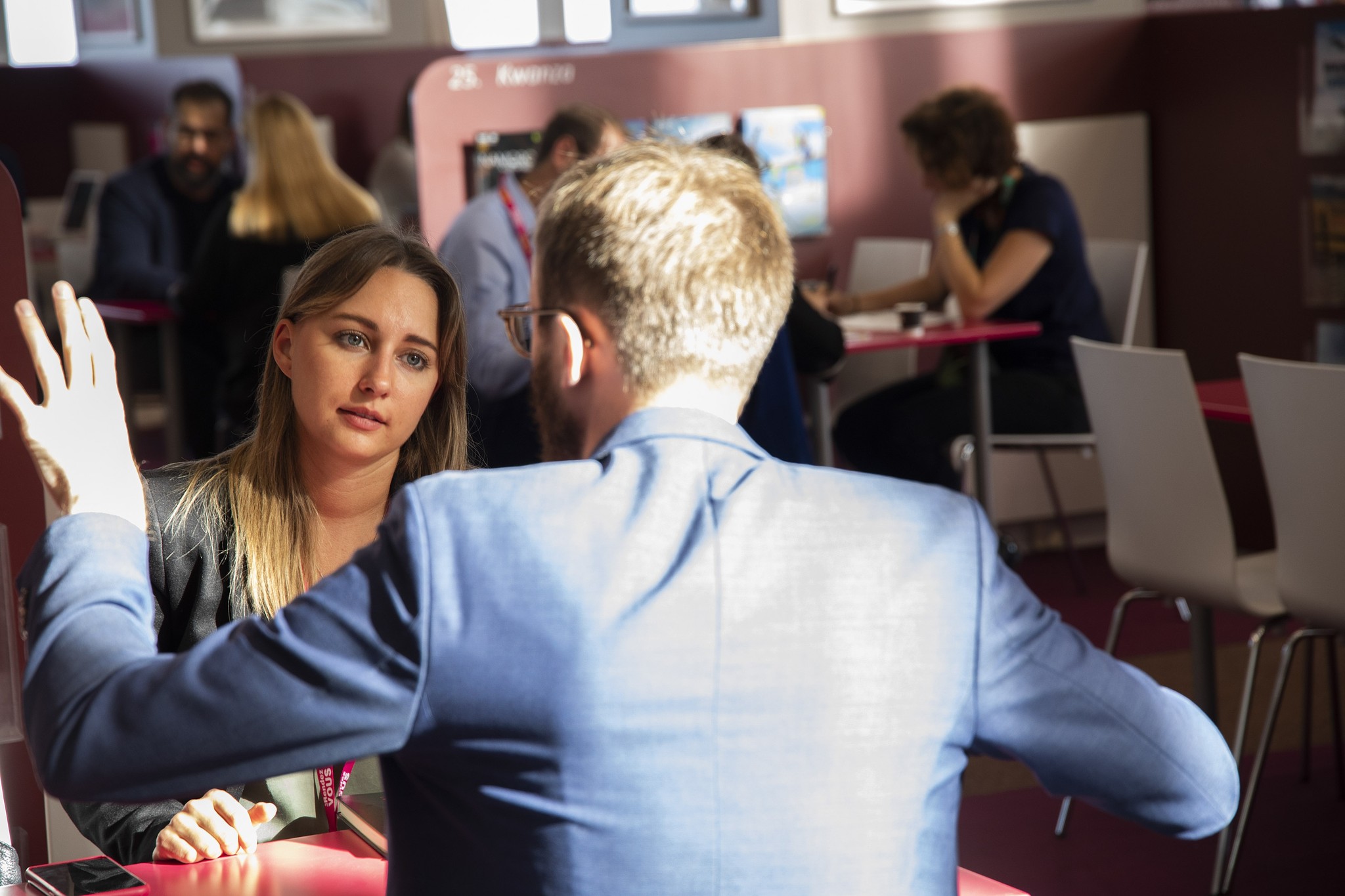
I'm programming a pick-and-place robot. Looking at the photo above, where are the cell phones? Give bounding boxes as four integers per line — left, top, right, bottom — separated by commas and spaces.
24, 855, 151, 896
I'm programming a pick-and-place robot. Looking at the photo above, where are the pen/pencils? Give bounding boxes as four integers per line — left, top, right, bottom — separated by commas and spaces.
821, 263, 835, 301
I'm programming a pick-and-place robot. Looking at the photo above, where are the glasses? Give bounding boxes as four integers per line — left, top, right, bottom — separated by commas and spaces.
496, 301, 594, 362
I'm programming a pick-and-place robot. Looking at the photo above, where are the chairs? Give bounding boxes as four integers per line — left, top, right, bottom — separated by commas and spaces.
802, 237, 935, 468
1052, 335, 1345, 896
945, 233, 1147, 592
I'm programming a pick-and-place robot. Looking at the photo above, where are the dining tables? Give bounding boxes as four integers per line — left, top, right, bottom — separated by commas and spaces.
0, 827, 1040, 896
812, 307, 1043, 536
91, 297, 192, 466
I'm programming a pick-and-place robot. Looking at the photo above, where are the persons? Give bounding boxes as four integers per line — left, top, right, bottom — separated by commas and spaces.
12, 221, 467, 863
177, 89, 386, 464
433, 103, 627, 467
688, 132, 848, 469
818, 82, 1128, 496
0, 137, 1242, 896
89, 78, 246, 460
